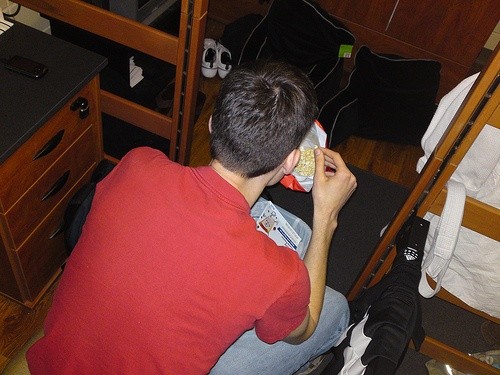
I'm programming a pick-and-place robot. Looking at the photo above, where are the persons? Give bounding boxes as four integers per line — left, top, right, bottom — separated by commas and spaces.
25, 60, 357, 375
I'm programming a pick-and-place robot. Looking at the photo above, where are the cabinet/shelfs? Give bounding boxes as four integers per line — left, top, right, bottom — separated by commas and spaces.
0, 15, 109, 310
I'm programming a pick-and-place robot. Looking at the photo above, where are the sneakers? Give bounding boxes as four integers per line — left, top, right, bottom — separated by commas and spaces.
200, 38, 217, 78
216, 42, 232, 79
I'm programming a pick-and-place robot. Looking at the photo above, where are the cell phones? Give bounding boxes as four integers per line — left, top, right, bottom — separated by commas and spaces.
5, 55, 47, 78
